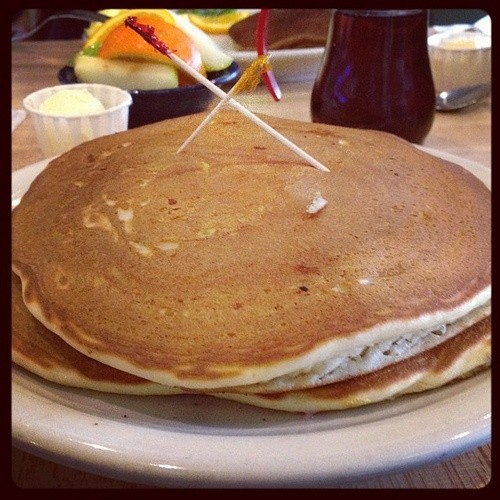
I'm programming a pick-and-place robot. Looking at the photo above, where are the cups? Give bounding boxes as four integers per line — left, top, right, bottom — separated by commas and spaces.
23, 83, 133, 158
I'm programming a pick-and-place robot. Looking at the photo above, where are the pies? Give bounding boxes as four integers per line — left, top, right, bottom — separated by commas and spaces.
11, 109, 491, 410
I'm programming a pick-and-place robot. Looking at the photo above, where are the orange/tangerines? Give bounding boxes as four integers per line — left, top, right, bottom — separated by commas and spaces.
83, 9, 207, 87
188, 11, 250, 34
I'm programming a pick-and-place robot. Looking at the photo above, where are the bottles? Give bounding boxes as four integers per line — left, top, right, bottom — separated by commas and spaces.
310, 8, 436, 144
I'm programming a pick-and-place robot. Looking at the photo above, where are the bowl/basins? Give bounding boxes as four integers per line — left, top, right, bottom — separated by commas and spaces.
423, 31, 491, 110
55, 61, 239, 126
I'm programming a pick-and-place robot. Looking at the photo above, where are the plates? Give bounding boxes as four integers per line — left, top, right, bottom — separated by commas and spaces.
223, 50, 324, 82
12, 151, 492, 486
